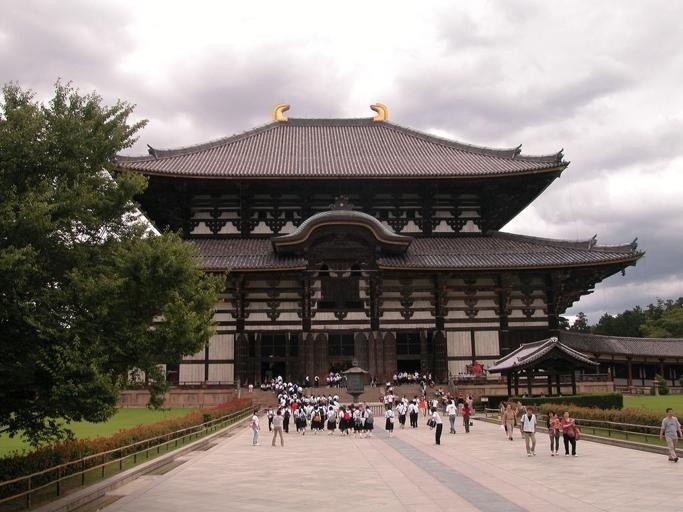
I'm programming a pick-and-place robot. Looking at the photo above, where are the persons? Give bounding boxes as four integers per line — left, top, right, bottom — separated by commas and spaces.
549, 412, 561, 434
557, 411, 579, 457
245, 368, 486, 449
515, 400, 528, 439
520, 406, 538, 457
659, 407, 683, 463
501, 403, 520, 443
545, 410, 561, 457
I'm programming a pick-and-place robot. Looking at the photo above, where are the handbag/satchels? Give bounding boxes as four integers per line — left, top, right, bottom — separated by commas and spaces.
344, 412, 349, 420
427, 419, 436, 428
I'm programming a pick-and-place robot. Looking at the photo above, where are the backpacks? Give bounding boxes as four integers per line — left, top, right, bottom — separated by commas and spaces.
355, 412, 361, 424
312, 410, 321, 422
366, 411, 374, 424
